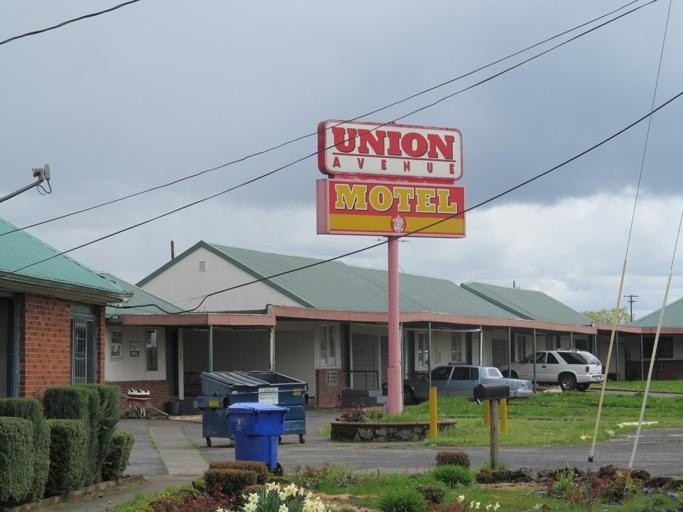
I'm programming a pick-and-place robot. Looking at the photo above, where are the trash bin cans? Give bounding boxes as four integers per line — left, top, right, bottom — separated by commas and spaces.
196, 368, 309, 447
224, 402, 290, 477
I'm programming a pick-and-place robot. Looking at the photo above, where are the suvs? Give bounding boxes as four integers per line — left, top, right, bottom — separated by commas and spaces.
497, 348, 607, 392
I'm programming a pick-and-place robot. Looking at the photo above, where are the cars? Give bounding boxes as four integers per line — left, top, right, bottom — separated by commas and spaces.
381, 361, 535, 407
